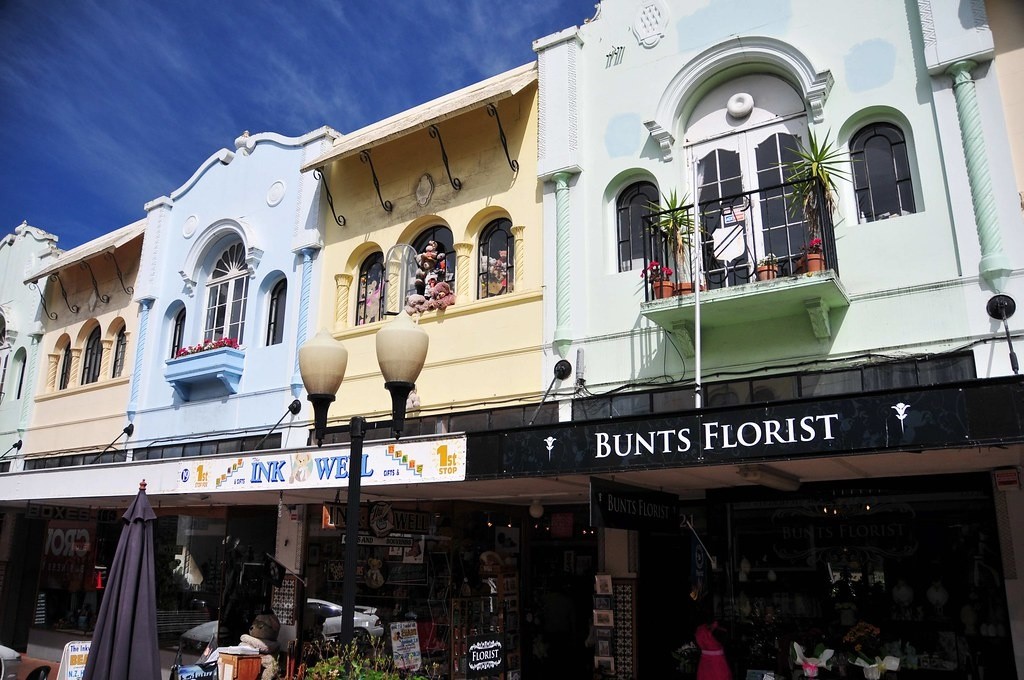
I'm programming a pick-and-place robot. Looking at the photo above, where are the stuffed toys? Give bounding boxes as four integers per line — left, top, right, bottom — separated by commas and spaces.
238, 610, 281, 680
404, 240, 456, 316
365, 275, 384, 324
481, 250, 508, 286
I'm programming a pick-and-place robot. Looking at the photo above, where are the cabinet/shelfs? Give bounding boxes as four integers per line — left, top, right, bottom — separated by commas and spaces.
451, 571, 521, 680
218, 653, 263, 680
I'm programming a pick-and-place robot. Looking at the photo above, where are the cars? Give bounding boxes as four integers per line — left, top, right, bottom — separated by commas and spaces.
180, 597, 384, 662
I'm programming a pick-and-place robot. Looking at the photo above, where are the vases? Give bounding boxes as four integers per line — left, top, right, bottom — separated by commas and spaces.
806, 254, 827, 272
757, 265, 778, 281
653, 281, 675, 299
164, 347, 245, 385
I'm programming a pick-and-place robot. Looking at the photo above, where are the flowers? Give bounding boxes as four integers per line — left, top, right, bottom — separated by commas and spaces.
640, 261, 673, 284
294, 635, 443, 680
757, 252, 778, 267
802, 238, 823, 272
175, 336, 240, 359
843, 622, 880, 665
671, 642, 700, 673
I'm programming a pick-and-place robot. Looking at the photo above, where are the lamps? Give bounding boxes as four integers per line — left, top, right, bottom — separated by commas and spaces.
529, 499, 544, 518
736, 464, 800, 491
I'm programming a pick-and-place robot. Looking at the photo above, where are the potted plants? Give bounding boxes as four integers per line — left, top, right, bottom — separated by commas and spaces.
640, 187, 713, 295
770, 124, 864, 273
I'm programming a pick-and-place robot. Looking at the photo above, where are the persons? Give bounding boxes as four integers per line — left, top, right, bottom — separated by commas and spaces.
695, 616, 733, 680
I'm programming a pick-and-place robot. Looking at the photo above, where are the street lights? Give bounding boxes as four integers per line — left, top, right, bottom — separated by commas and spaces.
297, 311, 429, 680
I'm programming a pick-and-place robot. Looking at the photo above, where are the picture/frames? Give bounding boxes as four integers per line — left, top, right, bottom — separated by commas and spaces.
595, 656, 614, 672
595, 575, 613, 594
593, 609, 614, 626
